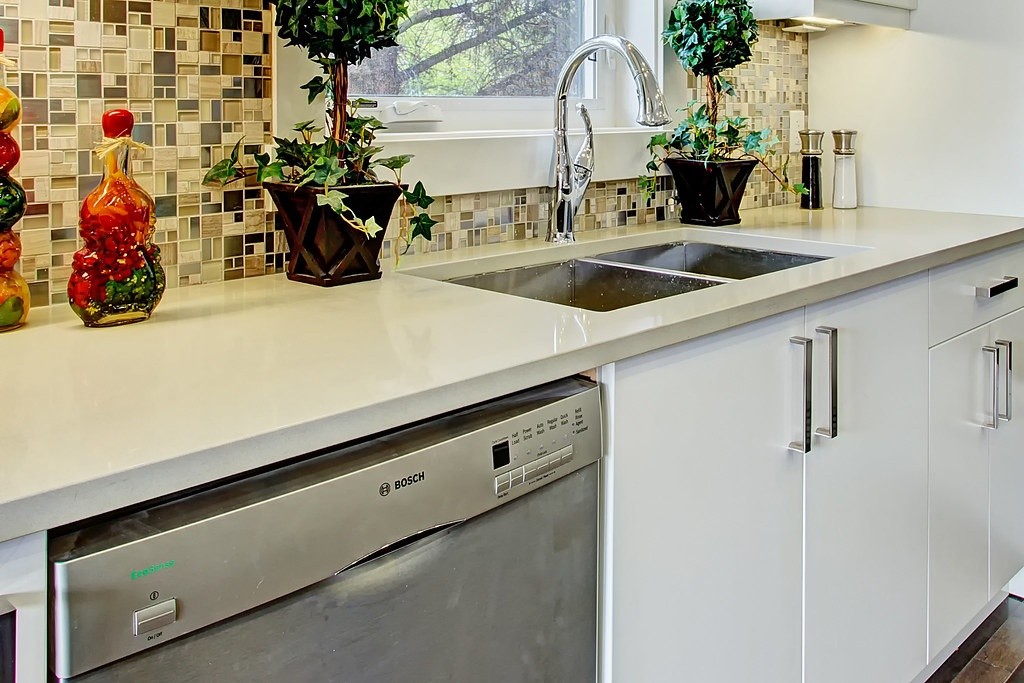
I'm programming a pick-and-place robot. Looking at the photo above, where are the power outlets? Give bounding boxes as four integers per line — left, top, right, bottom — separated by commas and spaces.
789, 110, 805, 153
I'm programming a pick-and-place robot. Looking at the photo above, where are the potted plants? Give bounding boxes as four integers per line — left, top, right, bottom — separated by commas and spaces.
637, 0, 810, 226
202, 1, 438, 289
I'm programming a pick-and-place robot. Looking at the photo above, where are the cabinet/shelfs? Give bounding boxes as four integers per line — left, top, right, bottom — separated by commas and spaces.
929, 241, 1024, 683
599, 271, 928, 682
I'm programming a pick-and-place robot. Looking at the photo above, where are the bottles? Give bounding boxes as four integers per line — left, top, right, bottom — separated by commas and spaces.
798, 128, 824, 209
66, 110, 168, 327
830, 129, 860, 210
0, 28, 31, 333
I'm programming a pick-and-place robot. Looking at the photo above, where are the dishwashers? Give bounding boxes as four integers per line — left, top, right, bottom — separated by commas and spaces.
48, 378, 602, 683
